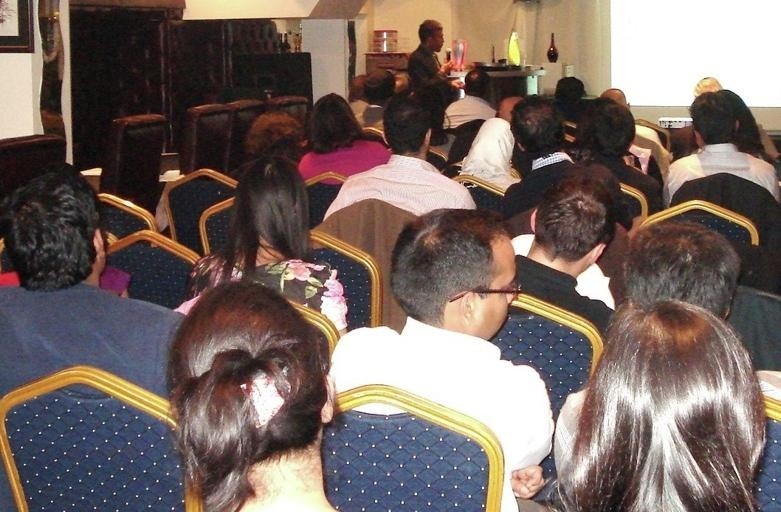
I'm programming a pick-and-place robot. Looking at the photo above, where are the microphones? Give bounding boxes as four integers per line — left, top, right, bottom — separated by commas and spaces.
445, 48, 452, 76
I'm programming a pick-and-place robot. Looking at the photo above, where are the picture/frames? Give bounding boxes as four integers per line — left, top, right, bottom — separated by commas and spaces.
0, 0, 34, 53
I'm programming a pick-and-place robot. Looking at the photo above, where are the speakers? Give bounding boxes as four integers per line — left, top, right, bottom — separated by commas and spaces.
232, 52, 313, 110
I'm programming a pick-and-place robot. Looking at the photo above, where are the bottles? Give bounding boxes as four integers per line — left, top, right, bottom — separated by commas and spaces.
233, 28, 301, 54
547, 34, 558, 64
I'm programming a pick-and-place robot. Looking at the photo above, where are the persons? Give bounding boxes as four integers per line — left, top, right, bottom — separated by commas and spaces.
0, 184, 133, 299
170, 279, 346, 510
227, 70, 781, 343
406, 19, 468, 94
549, 219, 781, 490
0, 158, 193, 511
562, 297, 768, 509
322, 205, 561, 512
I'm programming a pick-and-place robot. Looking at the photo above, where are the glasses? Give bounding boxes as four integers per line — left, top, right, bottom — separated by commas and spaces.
449, 271, 520, 306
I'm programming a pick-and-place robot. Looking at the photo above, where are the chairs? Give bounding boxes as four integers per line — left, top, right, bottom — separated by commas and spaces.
1, 95, 781, 512
